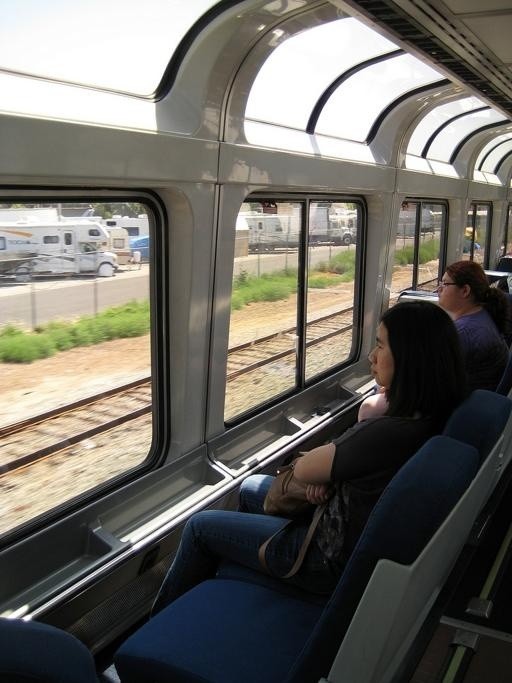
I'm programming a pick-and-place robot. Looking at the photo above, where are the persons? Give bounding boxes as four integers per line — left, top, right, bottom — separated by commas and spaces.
150, 300, 472, 619
357, 260, 511, 423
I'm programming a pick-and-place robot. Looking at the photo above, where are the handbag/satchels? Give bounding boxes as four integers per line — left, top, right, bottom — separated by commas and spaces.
262, 456, 331, 515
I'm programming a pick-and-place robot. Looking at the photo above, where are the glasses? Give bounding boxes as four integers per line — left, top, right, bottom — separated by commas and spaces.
438, 281, 458, 288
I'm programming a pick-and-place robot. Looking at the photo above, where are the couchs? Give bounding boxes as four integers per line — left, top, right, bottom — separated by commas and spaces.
212, 250, 511, 614
110, 435, 503, 680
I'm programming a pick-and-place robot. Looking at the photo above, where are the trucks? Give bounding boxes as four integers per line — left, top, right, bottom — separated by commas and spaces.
0, 206, 149, 281
246, 204, 437, 252
